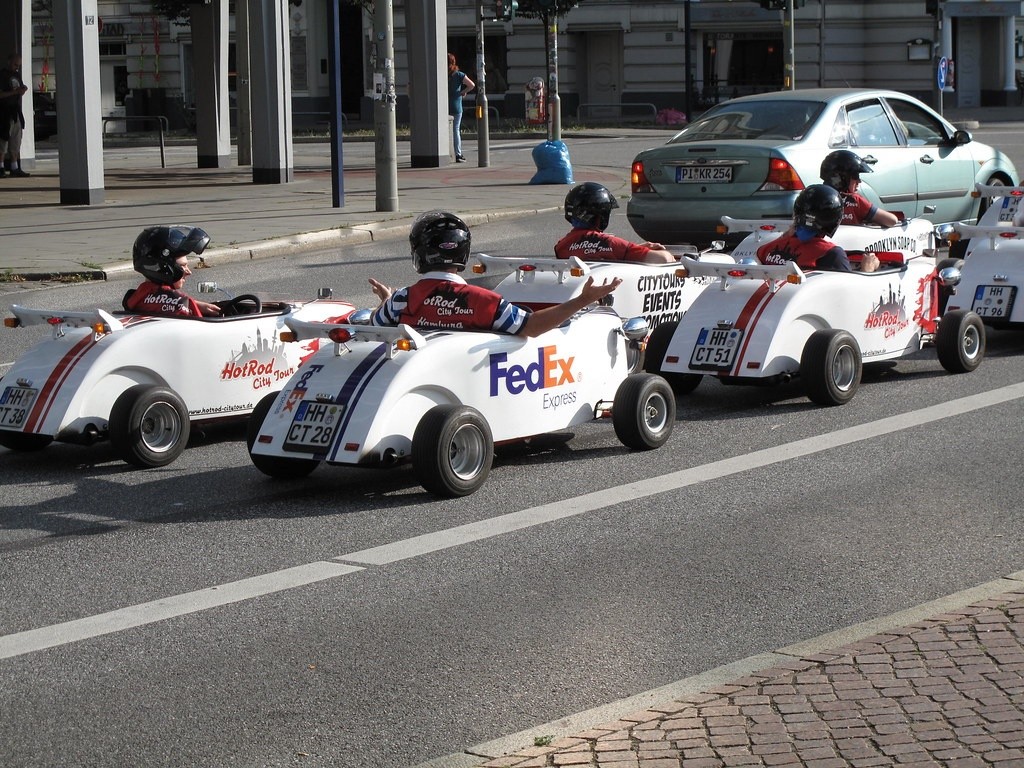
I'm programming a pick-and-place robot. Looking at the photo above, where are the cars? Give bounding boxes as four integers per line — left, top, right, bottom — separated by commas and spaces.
627, 88, 1019, 241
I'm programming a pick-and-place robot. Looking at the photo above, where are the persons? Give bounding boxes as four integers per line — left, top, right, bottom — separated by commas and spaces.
448, 53, 475, 163
369, 209, 623, 338
122, 226, 224, 319
753, 184, 880, 273
554, 182, 666, 262
820, 150, 898, 228
0, 54, 30, 177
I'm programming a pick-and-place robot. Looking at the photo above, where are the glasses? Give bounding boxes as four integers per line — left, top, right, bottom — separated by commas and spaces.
850, 172, 859, 181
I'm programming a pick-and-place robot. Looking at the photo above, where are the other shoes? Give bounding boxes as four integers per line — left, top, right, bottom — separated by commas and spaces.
456, 154, 467, 163
0, 167, 7, 178
9, 168, 31, 177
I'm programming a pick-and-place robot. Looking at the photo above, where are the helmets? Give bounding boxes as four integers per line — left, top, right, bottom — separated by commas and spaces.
821, 150, 875, 192
133, 227, 186, 285
794, 185, 844, 239
565, 182, 619, 228
410, 213, 472, 273
